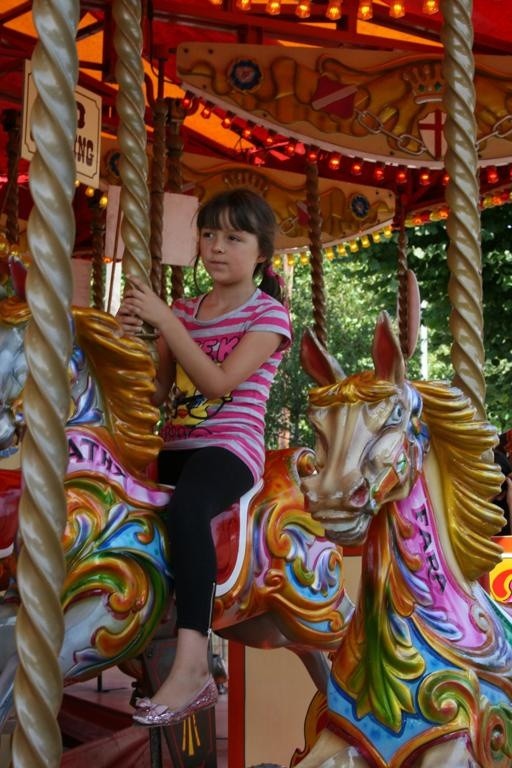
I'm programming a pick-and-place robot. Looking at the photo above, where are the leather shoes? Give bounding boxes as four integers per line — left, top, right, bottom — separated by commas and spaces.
132, 673, 221, 728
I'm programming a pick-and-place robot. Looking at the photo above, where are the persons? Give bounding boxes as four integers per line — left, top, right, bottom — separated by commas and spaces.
111, 188, 297, 727
489, 432, 512, 536
